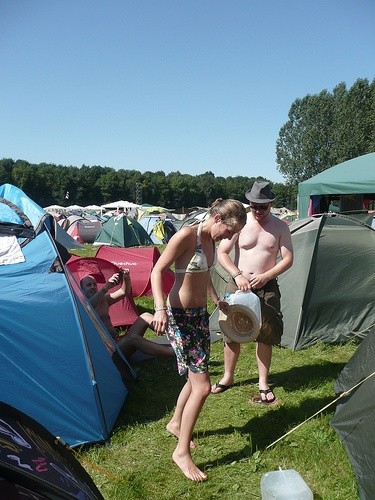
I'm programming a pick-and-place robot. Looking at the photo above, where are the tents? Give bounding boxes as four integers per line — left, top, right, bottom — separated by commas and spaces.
0, 151, 375, 500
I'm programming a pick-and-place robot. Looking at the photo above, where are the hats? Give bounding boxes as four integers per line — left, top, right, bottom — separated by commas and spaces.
218, 303, 260, 344
245, 180, 277, 204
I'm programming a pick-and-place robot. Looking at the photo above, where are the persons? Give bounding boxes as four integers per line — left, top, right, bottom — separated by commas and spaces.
80, 269, 176, 362
149, 198, 247, 481
211, 180, 294, 403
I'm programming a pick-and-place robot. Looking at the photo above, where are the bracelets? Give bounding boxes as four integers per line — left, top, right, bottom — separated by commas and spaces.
233, 271, 242, 279
154, 306, 167, 311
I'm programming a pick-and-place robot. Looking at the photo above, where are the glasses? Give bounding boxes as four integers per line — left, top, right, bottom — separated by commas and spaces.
250, 204, 269, 210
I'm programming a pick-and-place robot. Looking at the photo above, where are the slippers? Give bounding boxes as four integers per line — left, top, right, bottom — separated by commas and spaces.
258, 388, 275, 403
210, 378, 231, 394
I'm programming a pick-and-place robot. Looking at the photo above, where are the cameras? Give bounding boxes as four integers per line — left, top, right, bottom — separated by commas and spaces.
115, 271, 123, 285
